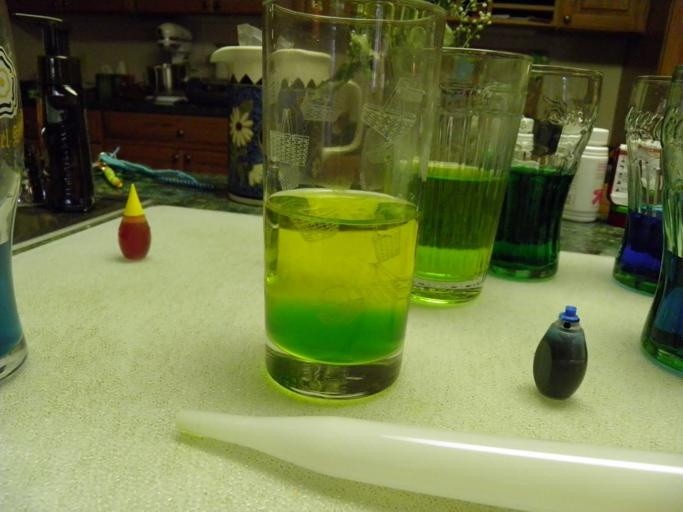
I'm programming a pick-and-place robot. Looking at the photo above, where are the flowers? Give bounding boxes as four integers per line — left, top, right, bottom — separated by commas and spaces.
311, 0, 492, 90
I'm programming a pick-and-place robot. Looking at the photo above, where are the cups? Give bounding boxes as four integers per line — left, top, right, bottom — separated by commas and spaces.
211, 37, 362, 207
260, 0, 445, 402
387, 46, 534, 308
483, 53, 604, 283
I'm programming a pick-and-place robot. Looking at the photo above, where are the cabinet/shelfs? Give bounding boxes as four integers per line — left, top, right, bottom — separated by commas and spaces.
431, 0, 673, 37
18, 108, 229, 183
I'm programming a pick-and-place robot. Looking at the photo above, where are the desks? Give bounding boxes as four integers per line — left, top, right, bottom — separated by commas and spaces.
0, 190, 683, 512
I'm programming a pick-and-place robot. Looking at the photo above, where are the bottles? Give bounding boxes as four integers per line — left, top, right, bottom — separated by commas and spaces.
612, 72, 671, 294
531, 303, 589, 399
116, 185, 152, 262
12, 24, 99, 212
566, 122, 608, 222
0, 46, 30, 386
643, 68, 683, 378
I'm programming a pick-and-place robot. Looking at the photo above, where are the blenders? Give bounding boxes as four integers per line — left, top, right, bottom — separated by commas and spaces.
146, 23, 192, 108
